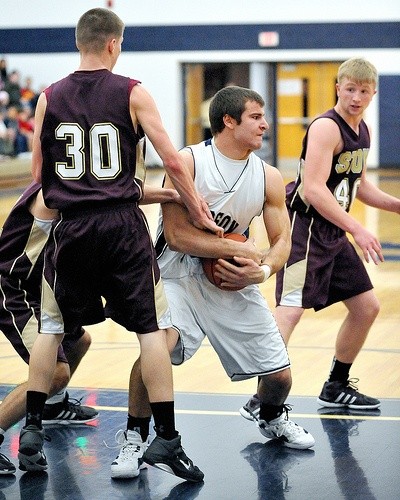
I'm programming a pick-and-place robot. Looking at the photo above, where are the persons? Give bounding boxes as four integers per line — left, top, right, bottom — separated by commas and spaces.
238, 56, 400, 426
107, 91, 315, 475
20, 10, 224, 481
0, 60, 205, 474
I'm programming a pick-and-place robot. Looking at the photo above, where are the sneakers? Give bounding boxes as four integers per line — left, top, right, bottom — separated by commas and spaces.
39, 393, 99, 422
143, 432, 204, 481
0, 434, 15, 475
42, 424, 97, 448
161, 482, 203, 500
317, 377, 380, 410
241, 440, 315, 480
110, 430, 148, 478
241, 395, 262, 421
256, 411, 315, 449
317, 408, 381, 436
18, 425, 49, 470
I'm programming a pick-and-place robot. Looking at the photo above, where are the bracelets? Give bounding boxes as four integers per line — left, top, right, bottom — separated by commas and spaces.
260, 265, 271, 283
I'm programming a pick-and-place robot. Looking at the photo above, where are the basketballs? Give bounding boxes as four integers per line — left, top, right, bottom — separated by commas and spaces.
202, 233, 256, 291
